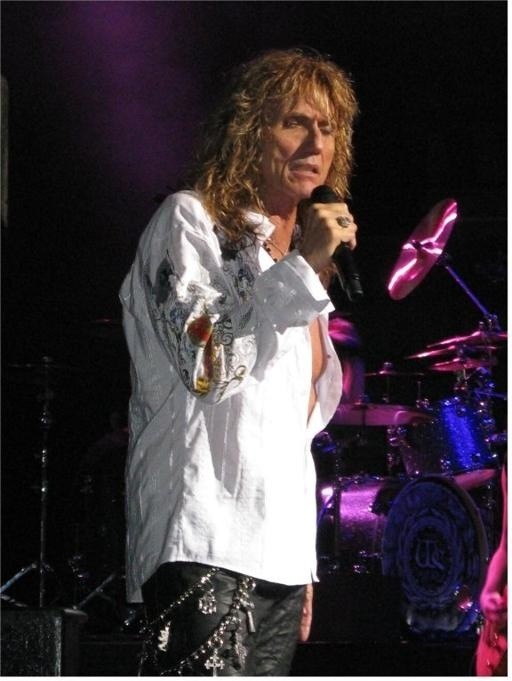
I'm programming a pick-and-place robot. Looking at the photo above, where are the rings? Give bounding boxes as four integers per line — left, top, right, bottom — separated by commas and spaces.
336, 215, 351, 228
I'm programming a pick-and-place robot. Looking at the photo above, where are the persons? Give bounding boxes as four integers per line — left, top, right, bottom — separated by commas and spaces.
327, 319, 363, 405
116, 46, 358, 676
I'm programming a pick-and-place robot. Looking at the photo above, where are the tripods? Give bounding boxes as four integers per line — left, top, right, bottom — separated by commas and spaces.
78, 524, 125, 607
0, 402, 64, 610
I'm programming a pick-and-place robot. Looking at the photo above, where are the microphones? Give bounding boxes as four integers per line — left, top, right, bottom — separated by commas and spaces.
311, 184, 366, 304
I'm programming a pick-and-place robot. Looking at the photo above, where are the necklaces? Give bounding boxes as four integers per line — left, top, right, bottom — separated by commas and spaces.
266, 238, 289, 257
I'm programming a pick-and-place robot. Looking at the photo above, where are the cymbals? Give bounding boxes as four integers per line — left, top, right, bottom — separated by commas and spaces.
408, 330, 507, 372
366, 369, 403, 377
337, 401, 410, 427
384, 199, 458, 299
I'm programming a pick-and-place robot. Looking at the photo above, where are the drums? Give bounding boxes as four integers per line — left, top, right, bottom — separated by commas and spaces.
434, 393, 498, 471
381, 475, 503, 645
315, 476, 390, 559
